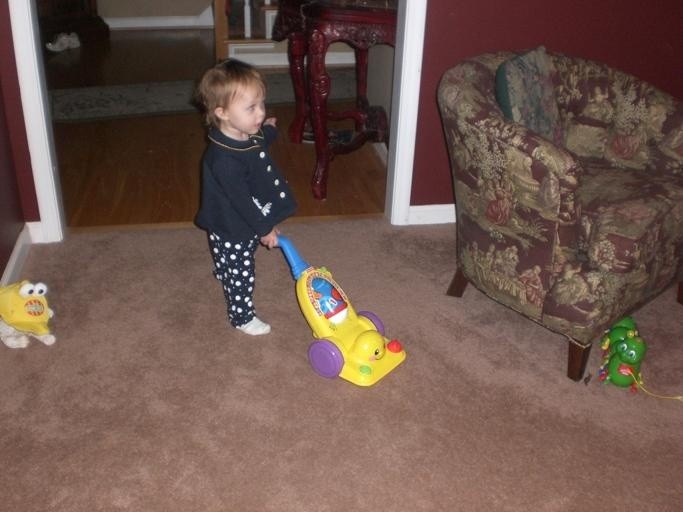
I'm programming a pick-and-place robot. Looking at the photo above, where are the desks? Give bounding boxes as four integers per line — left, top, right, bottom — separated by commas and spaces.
286, 6, 397, 202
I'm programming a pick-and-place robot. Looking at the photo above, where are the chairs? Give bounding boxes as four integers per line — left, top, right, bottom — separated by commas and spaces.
434, 51, 683, 383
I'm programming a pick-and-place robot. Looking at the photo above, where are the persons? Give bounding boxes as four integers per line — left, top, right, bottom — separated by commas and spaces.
194, 58, 296, 336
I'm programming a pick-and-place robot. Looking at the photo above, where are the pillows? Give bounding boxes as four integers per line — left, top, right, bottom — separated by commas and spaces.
494, 43, 563, 149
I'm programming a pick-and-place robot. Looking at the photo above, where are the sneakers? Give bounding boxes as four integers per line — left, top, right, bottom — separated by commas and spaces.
235, 315, 271, 336
